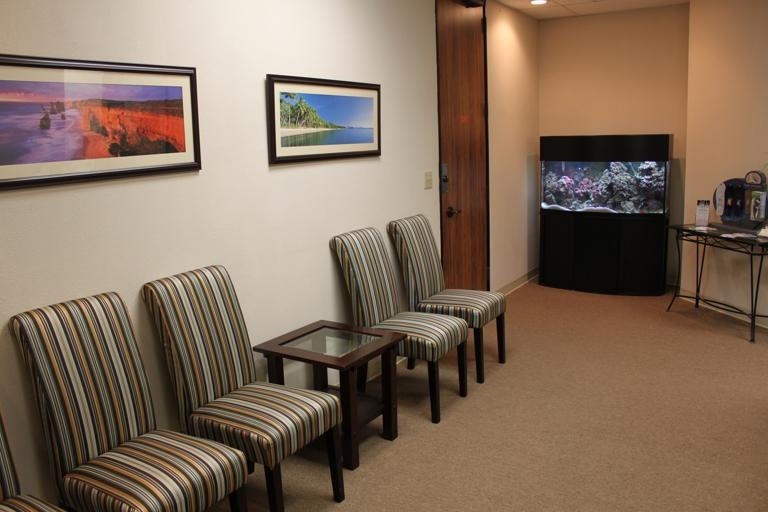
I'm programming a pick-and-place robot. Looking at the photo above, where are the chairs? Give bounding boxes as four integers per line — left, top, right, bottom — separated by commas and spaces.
142, 264, 346, 510
9, 291, 249, 512
330, 212, 509, 424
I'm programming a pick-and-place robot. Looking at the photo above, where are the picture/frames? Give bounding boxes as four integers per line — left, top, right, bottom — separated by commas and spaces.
264, 71, 383, 165
0, 50, 202, 188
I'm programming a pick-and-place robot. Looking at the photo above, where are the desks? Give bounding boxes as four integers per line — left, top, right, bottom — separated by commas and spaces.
666, 223, 768, 344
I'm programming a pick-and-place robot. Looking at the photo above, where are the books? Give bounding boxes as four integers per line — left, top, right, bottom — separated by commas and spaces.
749, 190, 766, 222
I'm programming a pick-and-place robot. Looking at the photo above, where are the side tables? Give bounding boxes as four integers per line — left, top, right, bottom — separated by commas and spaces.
251, 318, 408, 470
